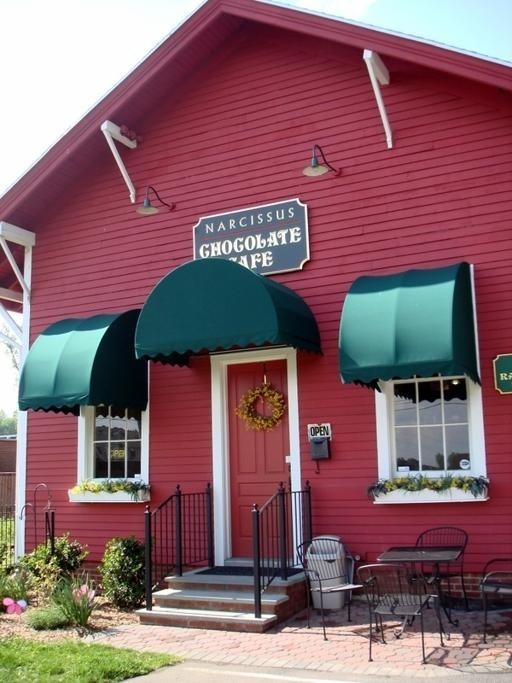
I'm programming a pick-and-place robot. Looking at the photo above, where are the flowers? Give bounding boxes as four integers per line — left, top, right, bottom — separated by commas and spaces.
367, 473, 491, 500
235, 382, 287, 430
71, 478, 151, 500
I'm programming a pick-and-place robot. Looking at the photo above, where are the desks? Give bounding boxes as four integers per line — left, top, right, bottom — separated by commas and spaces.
375, 544, 464, 637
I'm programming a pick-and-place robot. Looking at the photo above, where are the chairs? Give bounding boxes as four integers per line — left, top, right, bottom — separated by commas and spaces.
354, 563, 450, 666
478, 557, 512, 644
296, 537, 383, 640
407, 526, 468, 628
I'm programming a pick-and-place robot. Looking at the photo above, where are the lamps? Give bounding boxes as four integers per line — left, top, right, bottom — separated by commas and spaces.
301, 144, 342, 178
135, 185, 176, 215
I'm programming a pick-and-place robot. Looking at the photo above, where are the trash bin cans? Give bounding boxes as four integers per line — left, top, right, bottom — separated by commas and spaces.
305, 535, 345, 611
